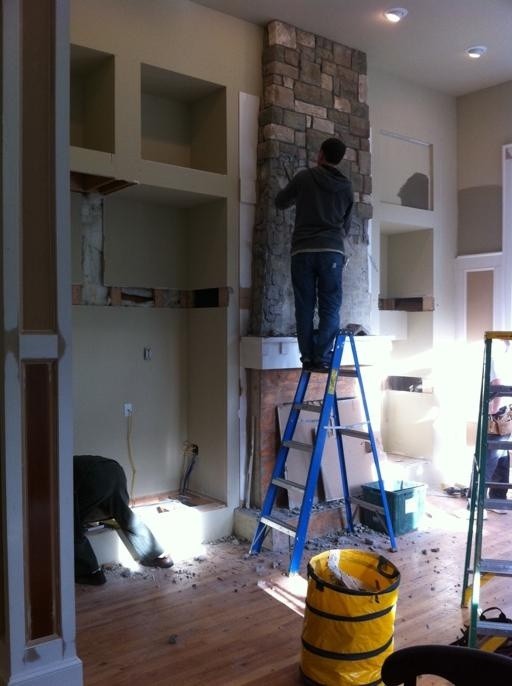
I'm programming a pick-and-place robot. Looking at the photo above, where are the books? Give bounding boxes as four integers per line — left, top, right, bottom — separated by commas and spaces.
348, 323, 369, 335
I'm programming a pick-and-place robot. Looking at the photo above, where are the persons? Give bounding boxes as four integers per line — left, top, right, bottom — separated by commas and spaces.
273, 137, 356, 371
467, 336, 512, 521
74, 453, 173, 587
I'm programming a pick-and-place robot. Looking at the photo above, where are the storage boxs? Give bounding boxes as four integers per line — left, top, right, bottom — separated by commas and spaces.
359, 479, 428, 536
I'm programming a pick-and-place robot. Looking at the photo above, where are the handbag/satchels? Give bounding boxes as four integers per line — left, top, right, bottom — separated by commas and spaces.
486, 406, 511, 438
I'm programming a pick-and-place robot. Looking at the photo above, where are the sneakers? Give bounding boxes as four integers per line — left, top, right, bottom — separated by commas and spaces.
139, 556, 173, 569
302, 359, 331, 372
78, 571, 106, 585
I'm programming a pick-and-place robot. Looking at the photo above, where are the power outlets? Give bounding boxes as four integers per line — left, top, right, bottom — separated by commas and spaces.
121, 402, 135, 418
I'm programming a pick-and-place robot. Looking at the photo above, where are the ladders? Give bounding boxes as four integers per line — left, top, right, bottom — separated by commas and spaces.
248, 329, 397, 578
460, 330, 512, 650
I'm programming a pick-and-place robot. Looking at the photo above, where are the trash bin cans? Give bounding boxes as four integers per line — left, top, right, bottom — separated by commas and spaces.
298, 549, 400, 686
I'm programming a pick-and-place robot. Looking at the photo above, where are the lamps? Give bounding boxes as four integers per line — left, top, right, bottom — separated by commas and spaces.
465, 45, 487, 60
384, 6, 409, 23
